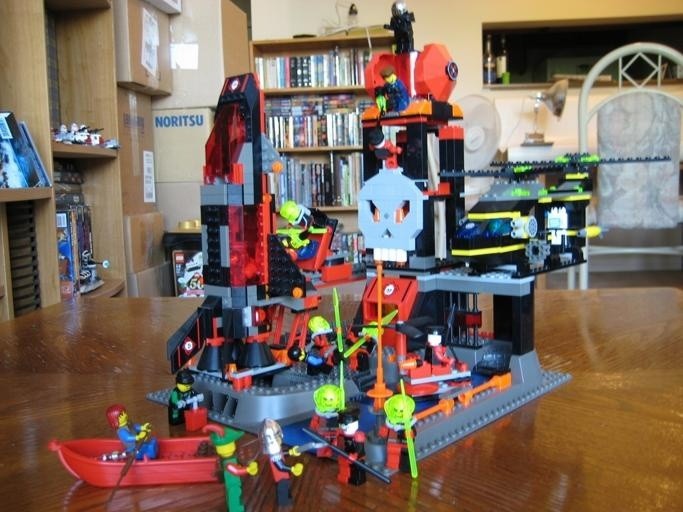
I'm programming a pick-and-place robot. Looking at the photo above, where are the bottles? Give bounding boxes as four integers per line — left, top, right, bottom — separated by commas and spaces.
496, 36, 508, 83
483, 34, 497, 85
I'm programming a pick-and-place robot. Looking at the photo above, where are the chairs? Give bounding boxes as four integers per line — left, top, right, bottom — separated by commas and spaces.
562, 39, 683, 292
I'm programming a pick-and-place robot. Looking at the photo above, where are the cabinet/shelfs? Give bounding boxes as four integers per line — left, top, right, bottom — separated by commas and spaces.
0, 2, 130, 324
249, 32, 402, 267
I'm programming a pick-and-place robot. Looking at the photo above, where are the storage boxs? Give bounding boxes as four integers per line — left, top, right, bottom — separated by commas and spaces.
114, 1, 250, 110
113, 85, 216, 296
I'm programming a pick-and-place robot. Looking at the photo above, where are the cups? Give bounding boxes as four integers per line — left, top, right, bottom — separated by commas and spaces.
177, 219, 202, 231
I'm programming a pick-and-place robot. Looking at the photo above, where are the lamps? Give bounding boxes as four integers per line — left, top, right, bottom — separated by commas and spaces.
518, 77, 571, 149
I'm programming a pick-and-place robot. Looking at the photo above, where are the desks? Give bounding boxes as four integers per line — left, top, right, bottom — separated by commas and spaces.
0, 291, 681, 511
504, 145, 566, 165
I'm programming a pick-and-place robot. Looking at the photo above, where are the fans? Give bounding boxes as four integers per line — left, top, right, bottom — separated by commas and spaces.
428, 92, 503, 179
